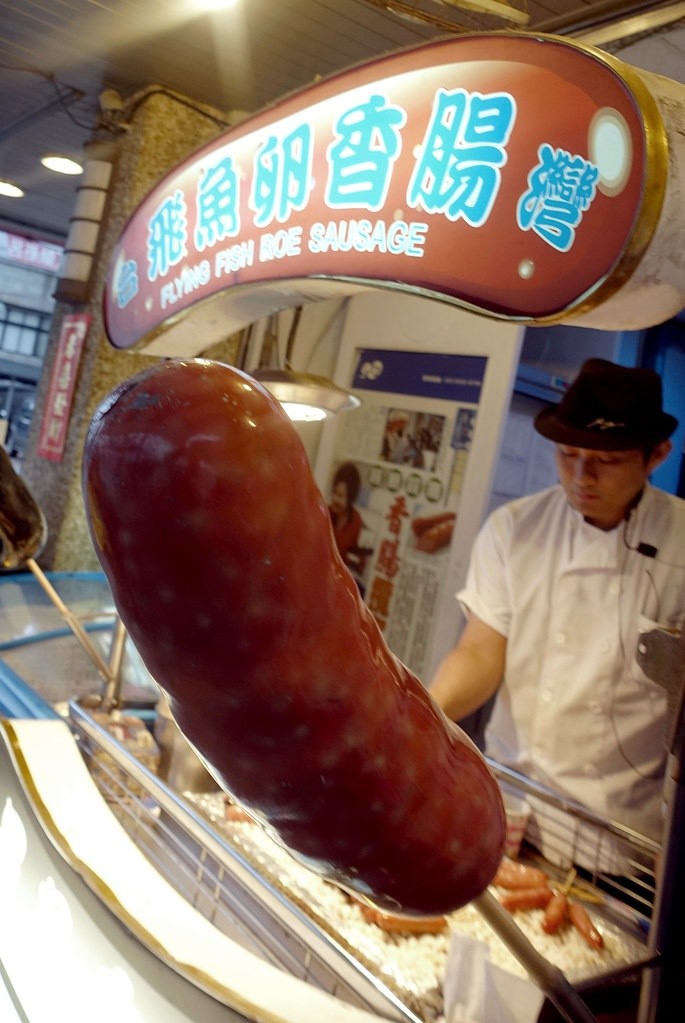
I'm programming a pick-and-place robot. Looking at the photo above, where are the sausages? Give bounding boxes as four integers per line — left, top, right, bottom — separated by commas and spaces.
487, 840, 616, 967
74, 357, 512, 921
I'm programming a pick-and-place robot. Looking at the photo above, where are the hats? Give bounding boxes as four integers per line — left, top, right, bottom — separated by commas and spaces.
534, 358, 678, 451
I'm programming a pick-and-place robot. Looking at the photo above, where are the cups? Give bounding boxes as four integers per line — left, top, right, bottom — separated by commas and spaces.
501, 792, 531, 862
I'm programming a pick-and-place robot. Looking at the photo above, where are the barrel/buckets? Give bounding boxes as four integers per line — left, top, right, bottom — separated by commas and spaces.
152, 702, 220, 797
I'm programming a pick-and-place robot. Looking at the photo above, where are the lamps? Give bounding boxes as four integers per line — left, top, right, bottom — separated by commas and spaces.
241, 304, 366, 428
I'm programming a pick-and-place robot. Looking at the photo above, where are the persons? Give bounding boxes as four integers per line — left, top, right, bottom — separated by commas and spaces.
325, 462, 360, 565
415, 360, 685, 915
